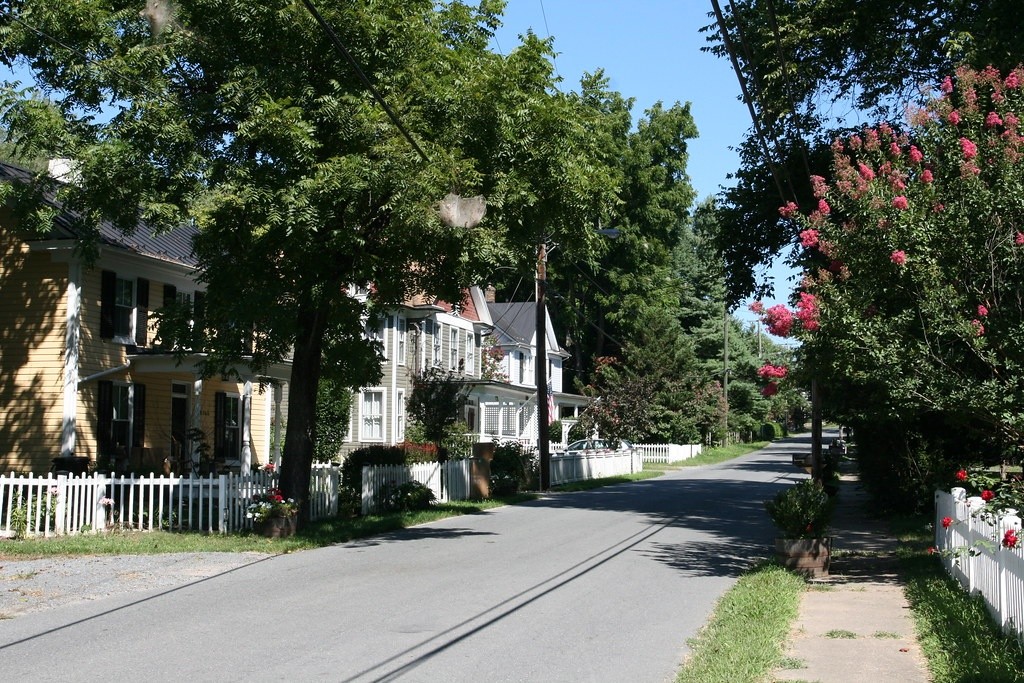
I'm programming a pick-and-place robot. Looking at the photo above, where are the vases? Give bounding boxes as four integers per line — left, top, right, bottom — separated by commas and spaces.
263, 518, 297, 537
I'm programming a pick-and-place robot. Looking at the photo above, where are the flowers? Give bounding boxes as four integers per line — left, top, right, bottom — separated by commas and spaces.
957, 470, 967, 481
1003, 530, 1017, 547
981, 489, 996, 502
942, 516, 952, 529
245, 489, 297, 522
928, 546, 933, 552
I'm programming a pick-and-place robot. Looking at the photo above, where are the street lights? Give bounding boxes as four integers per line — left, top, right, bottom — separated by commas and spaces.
535, 227, 620, 491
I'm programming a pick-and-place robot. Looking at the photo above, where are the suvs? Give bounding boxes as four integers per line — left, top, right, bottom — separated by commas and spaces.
555, 437, 633, 454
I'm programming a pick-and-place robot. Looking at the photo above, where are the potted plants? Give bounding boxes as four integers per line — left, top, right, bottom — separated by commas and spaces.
188, 428, 218, 478
388, 479, 435, 512
489, 439, 527, 497
763, 479, 836, 579
